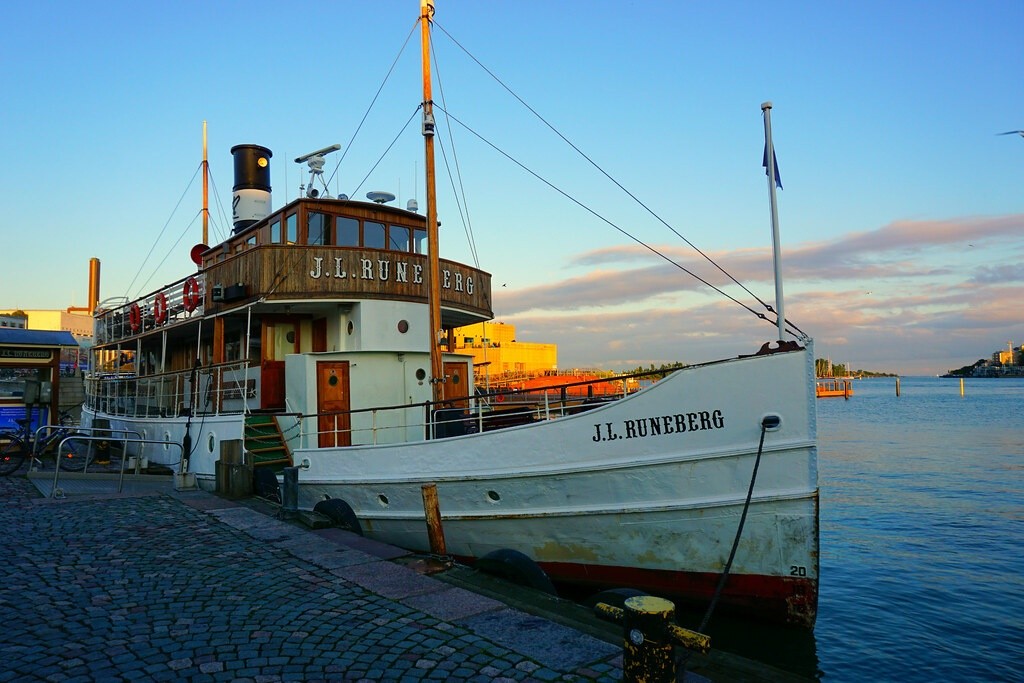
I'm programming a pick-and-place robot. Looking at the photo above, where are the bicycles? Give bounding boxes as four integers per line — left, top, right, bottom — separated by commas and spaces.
0, 400, 96, 476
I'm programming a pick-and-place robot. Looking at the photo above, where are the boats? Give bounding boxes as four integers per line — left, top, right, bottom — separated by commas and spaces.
78, 0, 821, 636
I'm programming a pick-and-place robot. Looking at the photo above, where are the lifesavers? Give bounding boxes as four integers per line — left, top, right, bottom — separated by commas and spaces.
154, 292, 166, 323
130, 305, 141, 331
182, 278, 199, 312
497, 395, 504, 402
